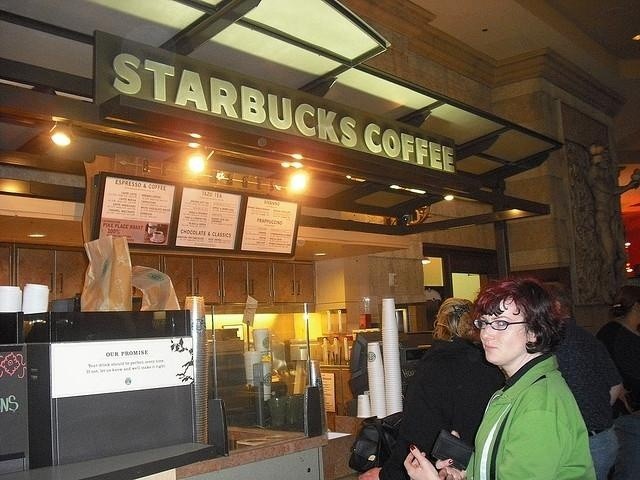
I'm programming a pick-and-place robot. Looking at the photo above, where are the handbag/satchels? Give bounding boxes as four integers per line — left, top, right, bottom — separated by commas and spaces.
348, 413, 402, 473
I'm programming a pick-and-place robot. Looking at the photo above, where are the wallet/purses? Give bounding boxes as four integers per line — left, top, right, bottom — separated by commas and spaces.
430, 430, 474, 470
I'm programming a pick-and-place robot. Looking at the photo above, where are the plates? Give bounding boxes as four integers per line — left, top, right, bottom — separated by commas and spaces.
150, 237, 166, 243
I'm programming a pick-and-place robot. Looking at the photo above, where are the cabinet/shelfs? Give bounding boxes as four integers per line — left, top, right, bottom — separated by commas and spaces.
0, 241, 318, 318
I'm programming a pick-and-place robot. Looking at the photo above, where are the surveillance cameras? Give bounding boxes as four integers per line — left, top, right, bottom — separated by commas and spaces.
401, 214, 413, 222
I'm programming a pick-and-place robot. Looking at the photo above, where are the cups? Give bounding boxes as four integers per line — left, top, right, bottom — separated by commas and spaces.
357, 299, 403, 419
303, 360, 327, 436
153, 231, 164, 241
183, 296, 208, 446
242, 327, 272, 401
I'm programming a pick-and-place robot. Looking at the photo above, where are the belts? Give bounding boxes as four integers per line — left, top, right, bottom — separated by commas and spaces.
588, 429, 604, 436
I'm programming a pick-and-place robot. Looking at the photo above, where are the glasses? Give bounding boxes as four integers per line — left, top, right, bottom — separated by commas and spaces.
474, 317, 528, 330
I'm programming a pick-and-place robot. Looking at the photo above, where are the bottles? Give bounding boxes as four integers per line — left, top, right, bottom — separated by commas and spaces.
359, 295, 372, 330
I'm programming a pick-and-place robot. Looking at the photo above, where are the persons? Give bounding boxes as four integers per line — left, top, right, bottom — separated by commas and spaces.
541, 281, 622, 480
403, 275, 598, 480
358, 299, 504, 480
597, 285, 640, 480
